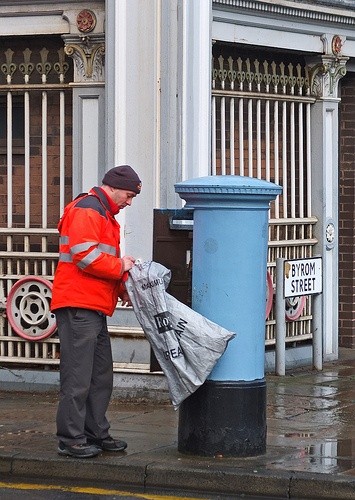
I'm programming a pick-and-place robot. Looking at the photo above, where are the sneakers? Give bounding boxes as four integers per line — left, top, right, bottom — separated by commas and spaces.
90, 436, 128, 452
57, 440, 102, 459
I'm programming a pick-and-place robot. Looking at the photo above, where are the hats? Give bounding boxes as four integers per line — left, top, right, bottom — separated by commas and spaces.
102, 164, 142, 194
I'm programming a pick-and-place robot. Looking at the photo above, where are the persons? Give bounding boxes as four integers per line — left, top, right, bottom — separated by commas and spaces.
49, 165, 143, 457
